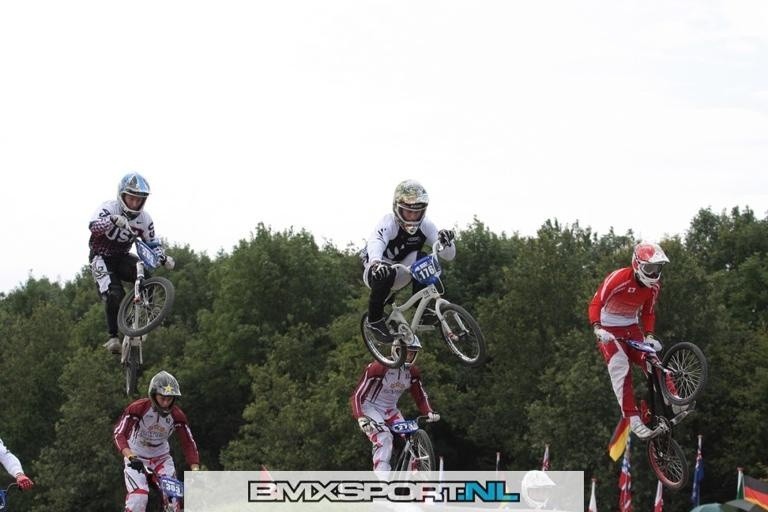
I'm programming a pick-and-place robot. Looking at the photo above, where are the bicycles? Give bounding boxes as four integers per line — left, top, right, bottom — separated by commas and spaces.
361, 240, 486, 368
363, 413, 448, 471
0, 480, 34, 512
127, 462, 206, 512
596, 335, 707, 491
110, 216, 175, 396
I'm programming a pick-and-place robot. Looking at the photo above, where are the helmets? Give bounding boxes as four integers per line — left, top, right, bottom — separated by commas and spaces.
631, 243, 671, 289
117, 173, 149, 219
393, 180, 429, 235
391, 333, 421, 367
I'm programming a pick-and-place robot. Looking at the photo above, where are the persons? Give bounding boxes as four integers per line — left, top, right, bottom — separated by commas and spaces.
90, 171, 175, 353
503, 470, 571, 511
0, 441, 35, 499
589, 241, 698, 439
112, 371, 200, 512
362, 180, 455, 344
351, 336, 441, 471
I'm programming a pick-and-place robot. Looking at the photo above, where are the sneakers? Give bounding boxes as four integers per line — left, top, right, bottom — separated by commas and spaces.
102, 336, 120, 350
630, 419, 652, 438
424, 313, 440, 326
367, 319, 393, 342
671, 400, 689, 414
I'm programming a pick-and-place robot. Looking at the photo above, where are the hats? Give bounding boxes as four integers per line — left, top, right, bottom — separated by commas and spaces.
149, 370, 181, 416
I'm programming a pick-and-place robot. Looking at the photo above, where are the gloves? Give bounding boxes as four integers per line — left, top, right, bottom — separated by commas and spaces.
427, 410, 440, 422
163, 256, 175, 270
437, 229, 456, 248
112, 214, 129, 229
128, 456, 144, 471
647, 335, 663, 352
371, 261, 391, 280
15, 474, 34, 490
595, 326, 614, 342
358, 417, 371, 432
